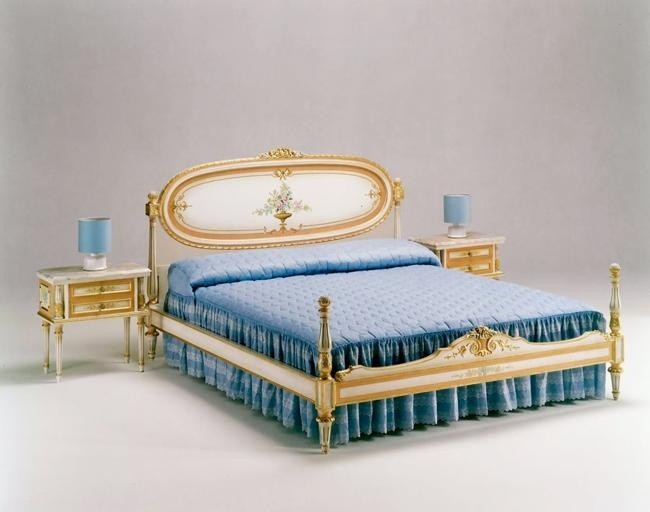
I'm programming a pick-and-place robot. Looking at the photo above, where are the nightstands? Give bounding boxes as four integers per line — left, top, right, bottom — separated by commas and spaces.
410, 233, 505, 282
36, 261, 152, 383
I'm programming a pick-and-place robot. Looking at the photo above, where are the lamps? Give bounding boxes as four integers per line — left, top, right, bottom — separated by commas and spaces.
77, 217, 113, 271
442, 194, 473, 237
144, 145, 625, 455
